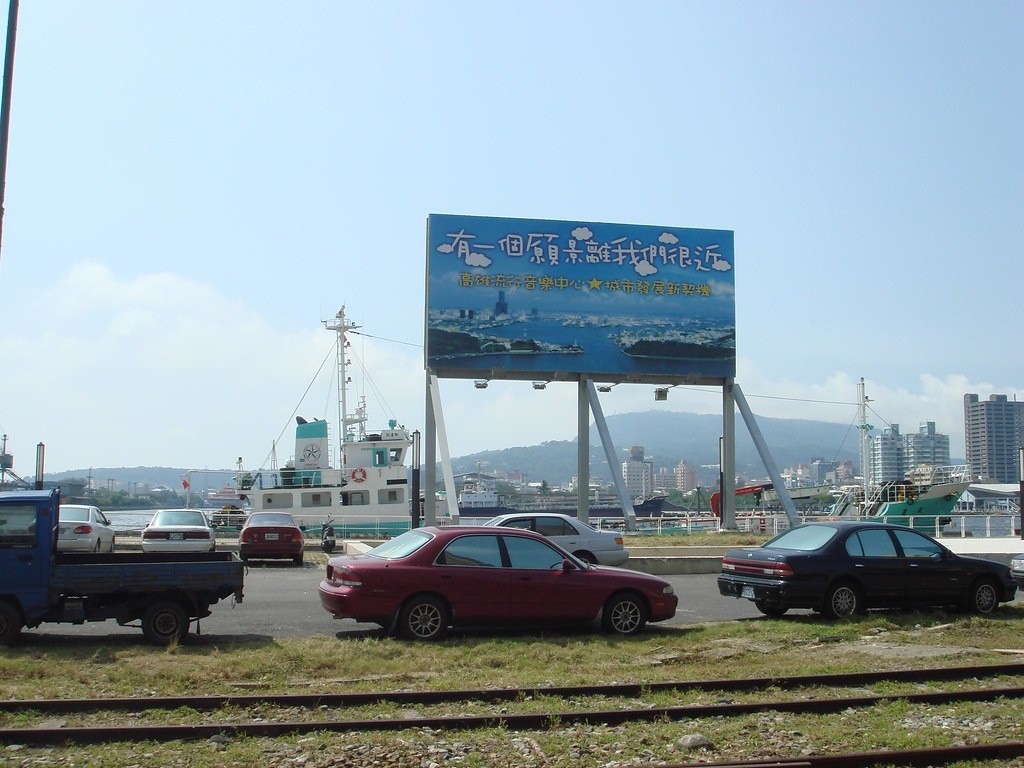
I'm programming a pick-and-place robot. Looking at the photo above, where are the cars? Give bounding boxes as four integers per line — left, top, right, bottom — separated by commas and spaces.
317, 523, 679, 643
717, 521, 1018, 621
141, 508, 219, 553
479, 512, 629, 568
29, 504, 116, 553
236, 511, 306, 566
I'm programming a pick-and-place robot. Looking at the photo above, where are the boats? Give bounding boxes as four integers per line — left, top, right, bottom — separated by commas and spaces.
208, 299, 973, 540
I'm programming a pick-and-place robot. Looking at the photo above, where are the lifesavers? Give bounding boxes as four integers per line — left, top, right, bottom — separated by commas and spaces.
946, 495, 951, 502
907, 498, 915, 505
353, 469, 366, 482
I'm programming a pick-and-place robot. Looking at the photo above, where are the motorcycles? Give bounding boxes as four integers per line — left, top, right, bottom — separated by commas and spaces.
319, 512, 336, 553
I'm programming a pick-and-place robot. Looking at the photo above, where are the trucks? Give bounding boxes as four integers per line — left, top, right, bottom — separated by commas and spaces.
0, 489, 249, 647
